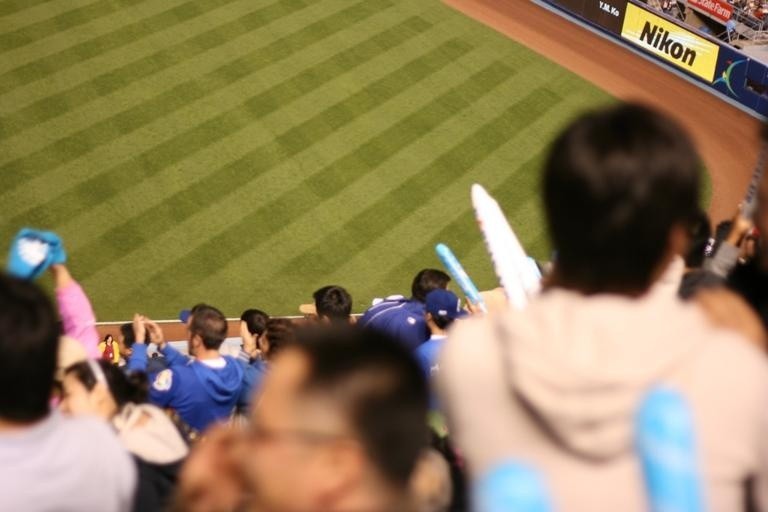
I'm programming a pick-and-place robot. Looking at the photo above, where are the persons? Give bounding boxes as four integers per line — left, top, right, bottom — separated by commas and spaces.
647, 0, 681, 20
685, 273, 766, 349
49, 264, 98, 415
116, 321, 150, 360
234, 318, 296, 413
187, 303, 210, 330
432, 102, 768, 512
713, 218, 747, 261
126, 307, 243, 439
164, 322, 452, 512
241, 309, 269, 349
685, 215, 751, 280
313, 285, 357, 325
0, 271, 140, 512
299, 303, 319, 324
59, 359, 191, 512
356, 268, 450, 360
414, 291, 469, 375
97, 333, 119, 365
727, 0, 768, 21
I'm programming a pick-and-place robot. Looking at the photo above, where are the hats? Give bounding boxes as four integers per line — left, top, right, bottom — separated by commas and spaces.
8, 228, 68, 284
179, 309, 191, 325
424, 289, 469, 321
